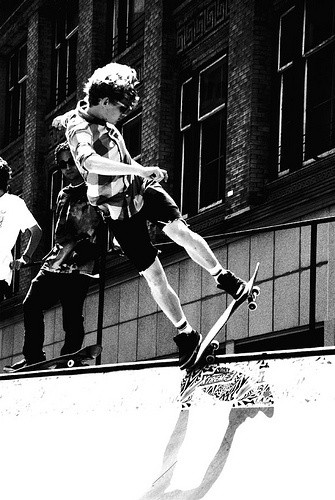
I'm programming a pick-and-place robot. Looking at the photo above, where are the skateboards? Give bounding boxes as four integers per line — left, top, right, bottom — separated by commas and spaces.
4, 344, 103, 373
186, 262, 261, 371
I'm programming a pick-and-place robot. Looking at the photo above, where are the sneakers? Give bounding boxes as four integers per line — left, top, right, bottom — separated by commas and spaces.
3, 359, 26, 370
217, 271, 248, 301
173, 329, 202, 370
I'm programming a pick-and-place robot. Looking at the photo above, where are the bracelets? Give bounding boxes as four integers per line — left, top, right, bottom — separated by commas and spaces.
23, 254, 31, 259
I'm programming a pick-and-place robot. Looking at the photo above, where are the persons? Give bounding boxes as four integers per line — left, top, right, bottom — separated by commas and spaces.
3, 141, 112, 374
51, 62, 246, 369
0, 157, 43, 303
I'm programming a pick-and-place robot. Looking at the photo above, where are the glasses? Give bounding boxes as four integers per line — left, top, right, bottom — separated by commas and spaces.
116, 98, 139, 117
59, 157, 75, 169
